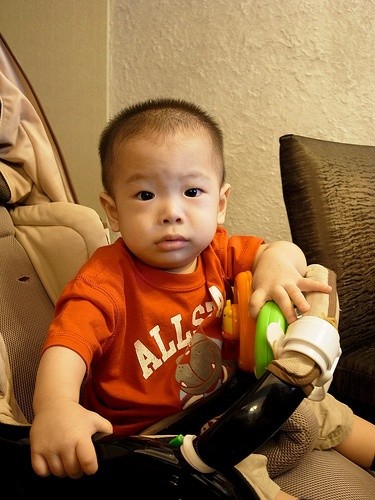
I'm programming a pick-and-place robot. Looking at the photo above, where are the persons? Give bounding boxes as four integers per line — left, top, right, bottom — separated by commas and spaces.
29, 98, 375, 481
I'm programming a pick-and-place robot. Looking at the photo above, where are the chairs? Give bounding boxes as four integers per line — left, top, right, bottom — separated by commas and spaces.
278, 135, 375, 427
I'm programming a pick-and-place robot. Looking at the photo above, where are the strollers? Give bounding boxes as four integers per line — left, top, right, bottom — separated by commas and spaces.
0, 33, 375, 500
